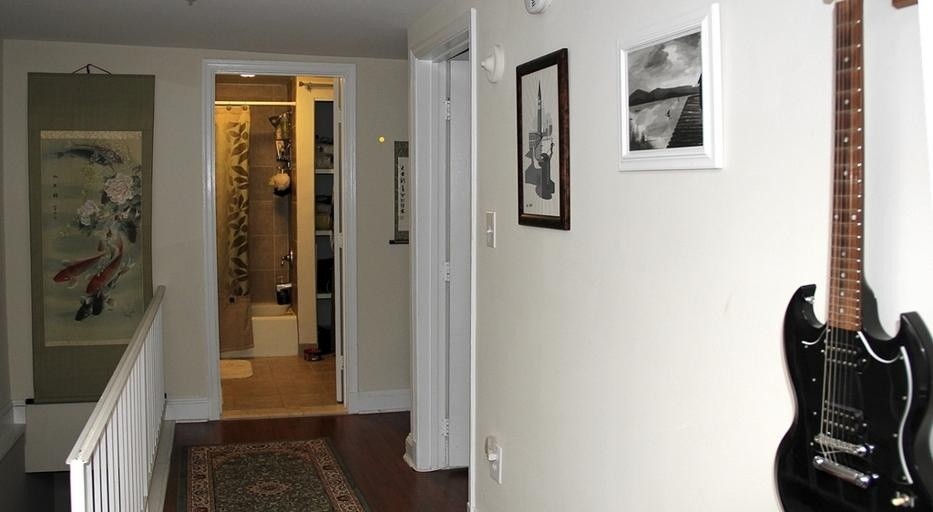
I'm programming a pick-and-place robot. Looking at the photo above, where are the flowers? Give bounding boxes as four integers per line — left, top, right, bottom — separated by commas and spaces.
61, 165, 144, 260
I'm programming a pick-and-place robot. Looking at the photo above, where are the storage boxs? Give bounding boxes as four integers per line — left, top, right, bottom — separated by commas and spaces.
315, 235, 334, 262
317, 299, 333, 330
315, 175, 333, 197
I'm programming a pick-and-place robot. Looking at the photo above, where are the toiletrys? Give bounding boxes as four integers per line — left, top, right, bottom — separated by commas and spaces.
280, 112, 290, 140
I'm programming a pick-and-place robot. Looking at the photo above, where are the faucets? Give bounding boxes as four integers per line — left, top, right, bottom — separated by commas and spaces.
285, 304, 294, 312
280, 249, 294, 267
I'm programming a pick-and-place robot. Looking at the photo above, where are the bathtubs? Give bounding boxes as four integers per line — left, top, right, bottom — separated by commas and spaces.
220, 302, 299, 357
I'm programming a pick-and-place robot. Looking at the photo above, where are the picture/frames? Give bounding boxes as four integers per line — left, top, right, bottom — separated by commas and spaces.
618, 3, 724, 173
515, 48, 572, 231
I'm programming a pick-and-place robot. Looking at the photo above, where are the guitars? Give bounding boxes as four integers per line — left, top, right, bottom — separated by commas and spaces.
774, 2, 933, 512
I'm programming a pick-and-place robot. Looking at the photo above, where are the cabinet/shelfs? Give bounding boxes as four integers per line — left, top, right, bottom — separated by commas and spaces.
315, 99, 336, 353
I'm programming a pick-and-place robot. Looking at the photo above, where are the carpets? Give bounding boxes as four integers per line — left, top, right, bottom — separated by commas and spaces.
220, 359, 253, 380
180, 435, 374, 512
